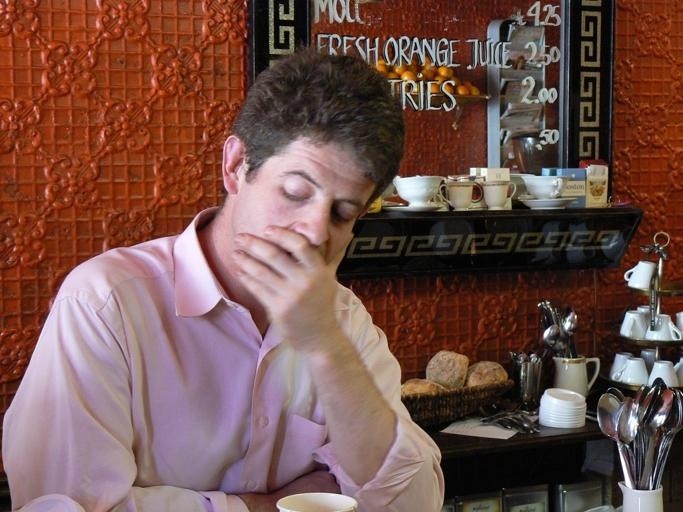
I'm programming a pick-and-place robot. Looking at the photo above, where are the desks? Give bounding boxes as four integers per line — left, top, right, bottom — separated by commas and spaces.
422, 417, 624, 509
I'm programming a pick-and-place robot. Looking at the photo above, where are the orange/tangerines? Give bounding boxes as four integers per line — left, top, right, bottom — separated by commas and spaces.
370, 56, 480, 98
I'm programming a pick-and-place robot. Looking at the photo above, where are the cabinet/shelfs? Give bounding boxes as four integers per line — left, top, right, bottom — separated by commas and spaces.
487, 18, 546, 168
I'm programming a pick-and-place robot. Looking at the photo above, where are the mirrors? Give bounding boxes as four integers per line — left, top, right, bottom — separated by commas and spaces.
245, 0, 643, 280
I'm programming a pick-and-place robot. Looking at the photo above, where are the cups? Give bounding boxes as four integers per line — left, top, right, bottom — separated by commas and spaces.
379, 161, 589, 217
273, 492, 355, 511
504, 363, 542, 410
549, 350, 602, 401
609, 302, 682, 393
620, 260, 661, 293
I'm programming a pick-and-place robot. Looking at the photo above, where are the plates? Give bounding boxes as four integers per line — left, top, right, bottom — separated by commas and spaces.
536, 385, 593, 431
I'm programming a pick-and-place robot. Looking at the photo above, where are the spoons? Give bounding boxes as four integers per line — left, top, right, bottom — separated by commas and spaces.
533, 298, 587, 358
591, 375, 683, 489
480, 402, 541, 435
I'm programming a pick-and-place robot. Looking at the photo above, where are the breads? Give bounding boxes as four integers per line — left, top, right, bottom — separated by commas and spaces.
398, 350, 509, 398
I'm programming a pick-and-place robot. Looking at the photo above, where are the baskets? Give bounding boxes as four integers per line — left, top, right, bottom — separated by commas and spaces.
401, 379, 514, 427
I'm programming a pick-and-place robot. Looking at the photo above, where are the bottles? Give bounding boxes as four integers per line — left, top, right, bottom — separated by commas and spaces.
616, 480, 664, 511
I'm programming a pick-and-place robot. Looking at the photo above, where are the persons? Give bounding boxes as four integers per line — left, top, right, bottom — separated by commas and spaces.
1, 49, 446, 512
